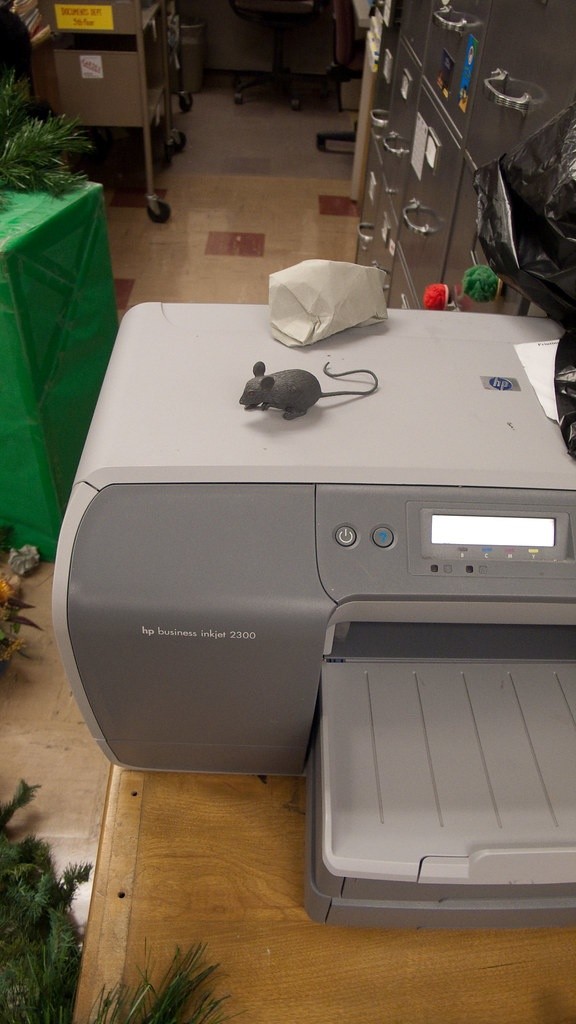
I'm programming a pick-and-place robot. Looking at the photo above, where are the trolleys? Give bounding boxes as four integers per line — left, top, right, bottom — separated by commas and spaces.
33, 0, 193, 224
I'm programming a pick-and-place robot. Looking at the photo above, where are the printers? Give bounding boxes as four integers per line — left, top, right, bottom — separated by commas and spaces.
51, 300, 576, 928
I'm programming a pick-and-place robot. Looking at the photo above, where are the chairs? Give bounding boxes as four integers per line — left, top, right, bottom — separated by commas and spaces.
315, 0, 365, 151
229, 0, 321, 111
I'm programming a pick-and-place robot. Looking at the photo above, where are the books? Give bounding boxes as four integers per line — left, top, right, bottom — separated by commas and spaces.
11, 0, 50, 43
